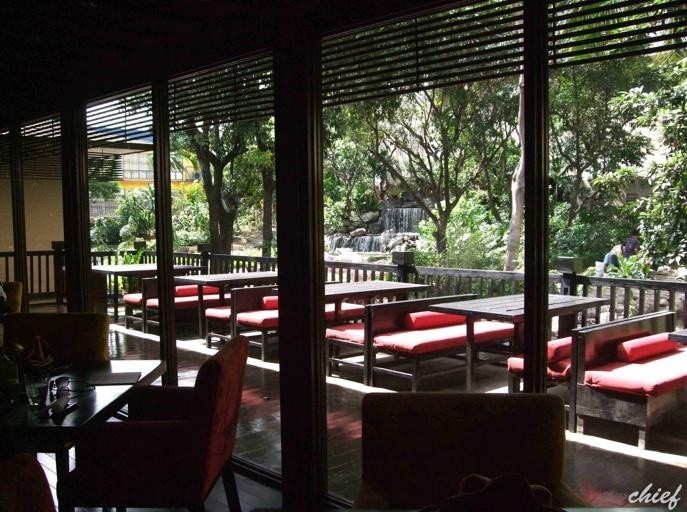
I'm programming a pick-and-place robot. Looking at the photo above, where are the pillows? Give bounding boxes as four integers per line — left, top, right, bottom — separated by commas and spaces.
458, 474, 553, 508
438, 471, 561, 512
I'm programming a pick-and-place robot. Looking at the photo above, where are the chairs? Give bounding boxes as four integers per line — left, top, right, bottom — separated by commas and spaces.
0, 452, 56, 512
3, 312, 111, 362
350, 390, 592, 512
1, 281, 24, 316
56, 335, 249, 512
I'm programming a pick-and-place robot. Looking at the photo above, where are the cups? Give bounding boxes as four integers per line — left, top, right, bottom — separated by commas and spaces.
24, 371, 49, 405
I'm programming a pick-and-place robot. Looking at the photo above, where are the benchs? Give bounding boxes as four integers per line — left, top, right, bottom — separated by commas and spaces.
90, 263, 687, 449
